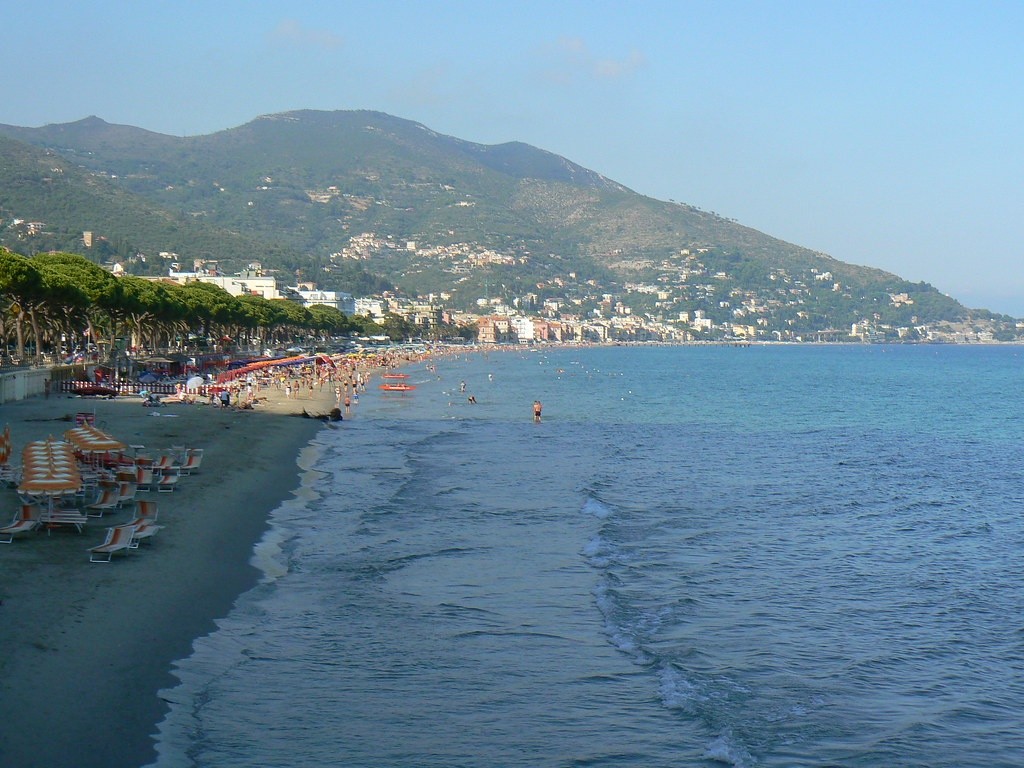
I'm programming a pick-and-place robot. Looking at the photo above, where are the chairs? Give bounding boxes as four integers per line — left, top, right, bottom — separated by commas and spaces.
0, 445, 204, 544
105, 500, 166, 548
86, 526, 134, 563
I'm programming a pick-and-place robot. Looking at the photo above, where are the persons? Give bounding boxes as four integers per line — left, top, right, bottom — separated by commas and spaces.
180, 393, 195, 403
219, 342, 527, 413
175, 381, 182, 397
534, 401, 542, 421
144, 392, 166, 408
163, 371, 168, 377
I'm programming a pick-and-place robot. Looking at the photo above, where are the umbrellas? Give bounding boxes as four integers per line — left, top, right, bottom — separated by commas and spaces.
19, 439, 80, 533
140, 374, 156, 384
66, 420, 127, 471
0, 426, 12, 463
187, 377, 204, 389
